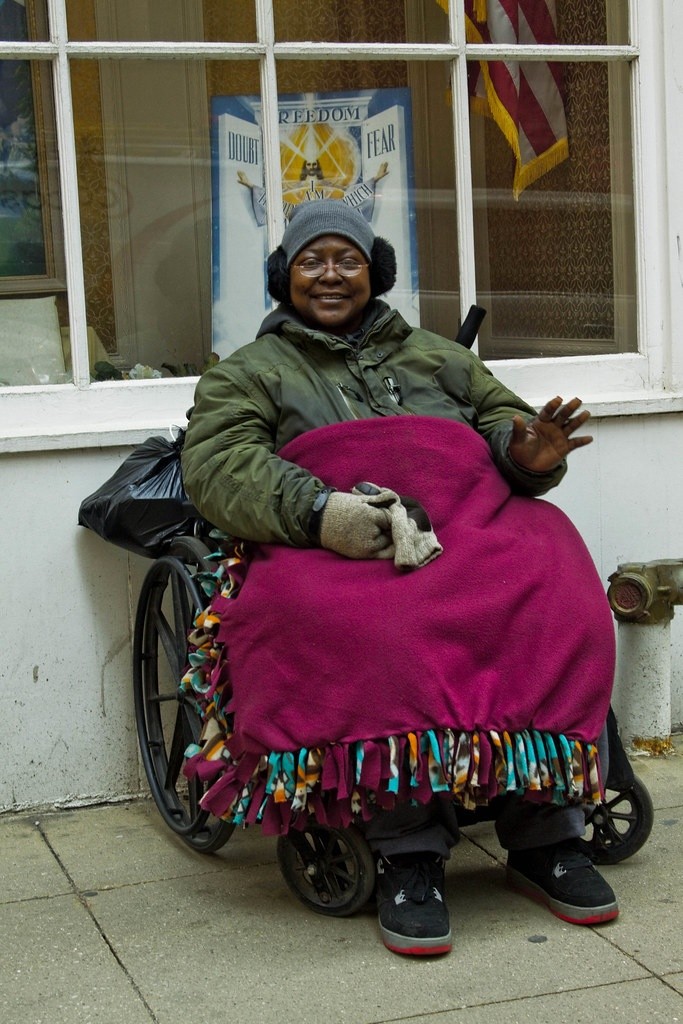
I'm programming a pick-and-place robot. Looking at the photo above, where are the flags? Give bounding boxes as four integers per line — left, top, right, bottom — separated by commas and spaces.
435, 0, 570, 202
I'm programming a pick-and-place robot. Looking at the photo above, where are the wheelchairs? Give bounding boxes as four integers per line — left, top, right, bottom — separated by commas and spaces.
132, 305, 655, 916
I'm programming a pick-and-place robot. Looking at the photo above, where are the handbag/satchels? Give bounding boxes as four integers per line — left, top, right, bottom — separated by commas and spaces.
77, 424, 234, 565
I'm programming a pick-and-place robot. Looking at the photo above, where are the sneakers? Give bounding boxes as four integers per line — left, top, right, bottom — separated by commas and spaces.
377, 848, 454, 954
506, 836, 619, 925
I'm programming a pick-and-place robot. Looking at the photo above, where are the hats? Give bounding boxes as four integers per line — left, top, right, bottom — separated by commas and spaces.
267, 200, 396, 309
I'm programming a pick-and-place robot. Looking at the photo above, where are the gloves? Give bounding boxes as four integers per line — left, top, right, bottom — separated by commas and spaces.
319, 489, 398, 559
352, 481, 444, 572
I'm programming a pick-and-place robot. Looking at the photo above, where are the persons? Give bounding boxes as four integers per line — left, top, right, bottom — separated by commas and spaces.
237, 154, 389, 226
180, 200, 619, 956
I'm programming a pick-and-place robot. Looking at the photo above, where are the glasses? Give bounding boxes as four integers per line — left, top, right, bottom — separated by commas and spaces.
290, 259, 369, 278
